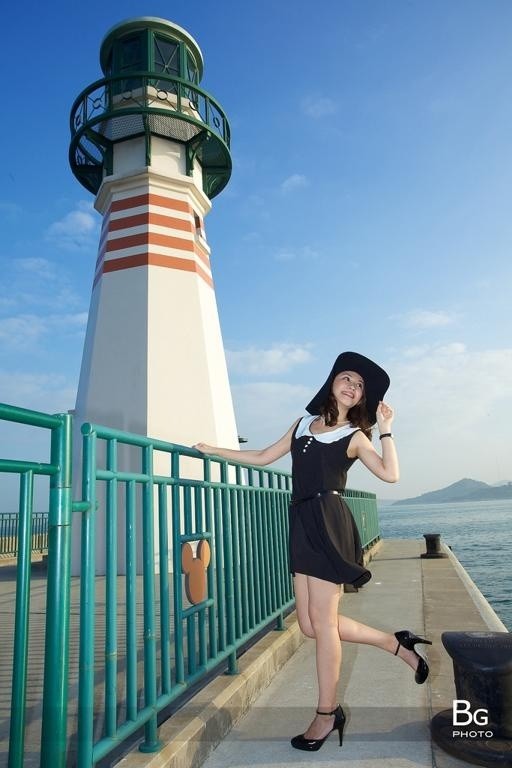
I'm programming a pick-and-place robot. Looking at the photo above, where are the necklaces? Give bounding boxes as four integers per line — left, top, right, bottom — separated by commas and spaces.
319, 415, 352, 426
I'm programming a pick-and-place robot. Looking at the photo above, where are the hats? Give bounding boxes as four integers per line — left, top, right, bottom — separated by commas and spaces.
306, 352, 389, 426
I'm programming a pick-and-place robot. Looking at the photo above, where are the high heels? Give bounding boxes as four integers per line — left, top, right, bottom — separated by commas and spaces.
394, 630, 433, 684
290, 705, 344, 751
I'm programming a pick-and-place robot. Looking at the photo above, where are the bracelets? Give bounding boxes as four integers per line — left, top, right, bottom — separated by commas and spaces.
378, 432, 394, 440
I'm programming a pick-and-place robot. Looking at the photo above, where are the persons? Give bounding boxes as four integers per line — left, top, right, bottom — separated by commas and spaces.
192, 350, 433, 754
